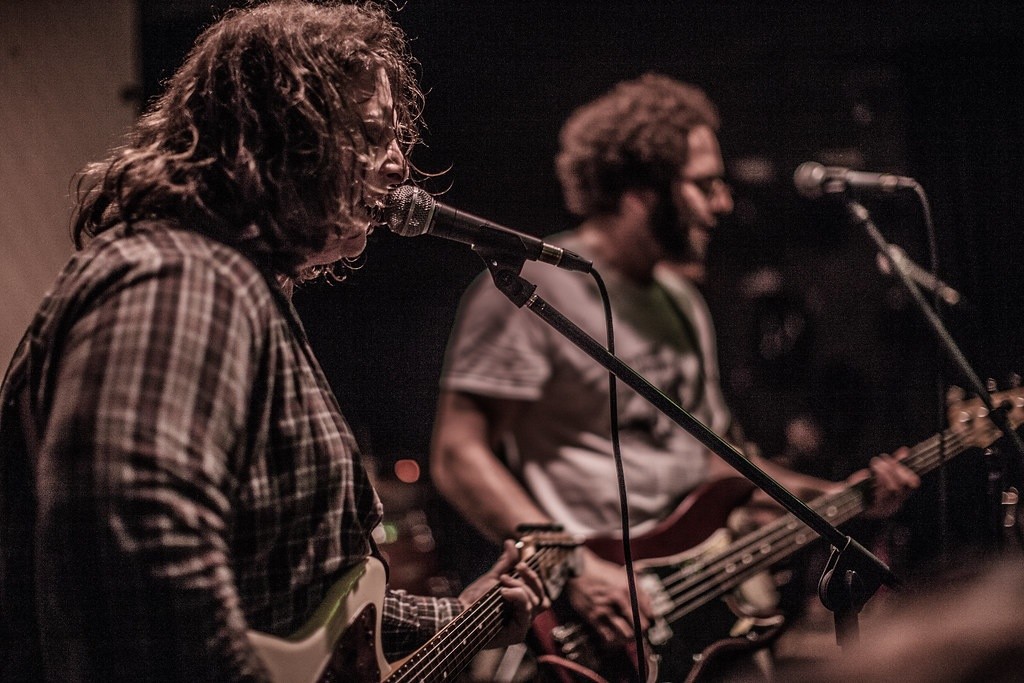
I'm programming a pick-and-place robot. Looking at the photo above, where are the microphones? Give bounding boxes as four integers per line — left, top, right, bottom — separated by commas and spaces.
385, 185, 593, 275
794, 161, 916, 200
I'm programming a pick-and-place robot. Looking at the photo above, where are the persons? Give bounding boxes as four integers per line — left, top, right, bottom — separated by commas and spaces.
431, 74, 921, 683
0, 0, 551, 683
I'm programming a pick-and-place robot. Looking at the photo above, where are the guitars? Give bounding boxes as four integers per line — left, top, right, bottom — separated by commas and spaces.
526, 372, 1024, 683
247, 521, 577, 683
